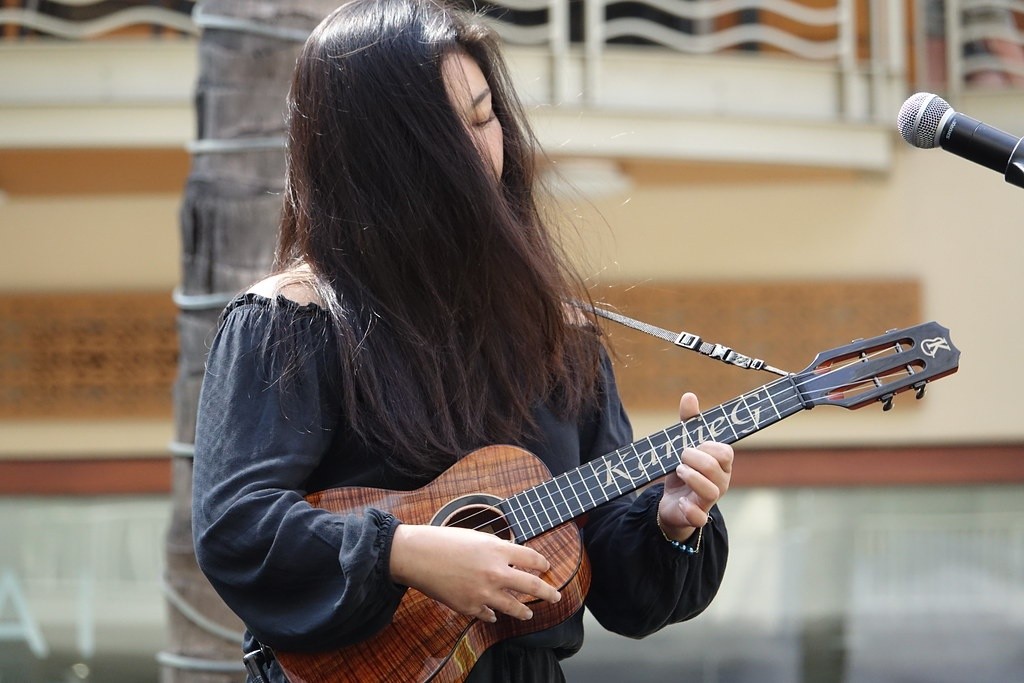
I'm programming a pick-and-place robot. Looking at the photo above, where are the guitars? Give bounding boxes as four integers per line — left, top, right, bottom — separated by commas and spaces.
266, 318, 962, 682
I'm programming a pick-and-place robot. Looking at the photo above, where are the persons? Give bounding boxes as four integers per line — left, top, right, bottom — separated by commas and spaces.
190, 1, 735, 683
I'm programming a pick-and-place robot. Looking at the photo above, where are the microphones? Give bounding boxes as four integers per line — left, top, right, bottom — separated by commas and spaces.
896, 91, 1024, 189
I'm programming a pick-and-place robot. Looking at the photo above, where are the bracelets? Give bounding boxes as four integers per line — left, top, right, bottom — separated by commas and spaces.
656, 503, 713, 553
